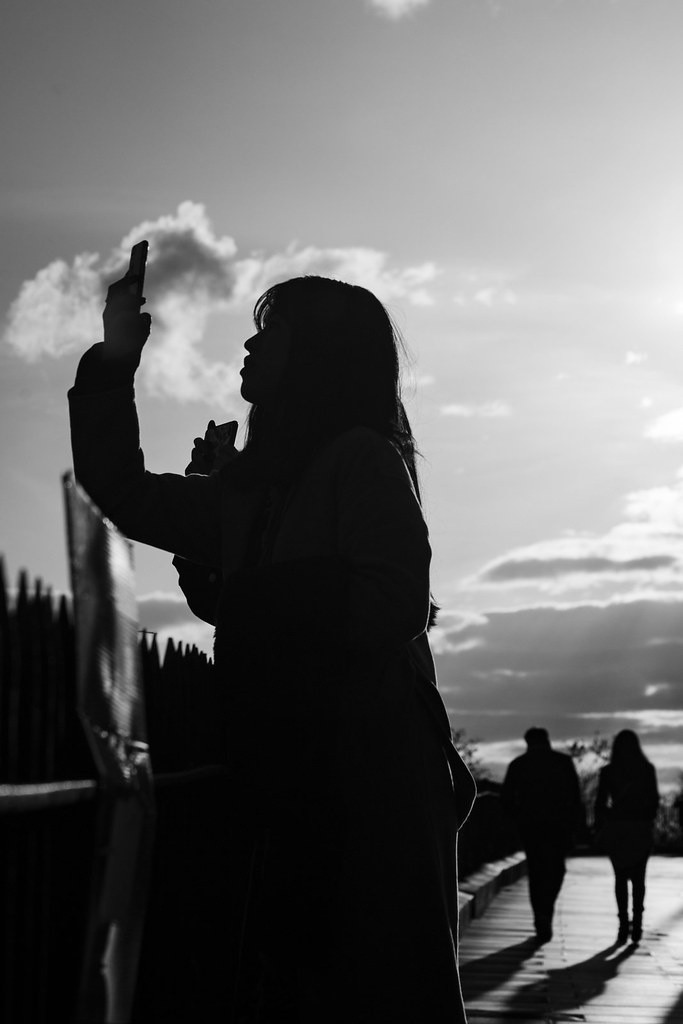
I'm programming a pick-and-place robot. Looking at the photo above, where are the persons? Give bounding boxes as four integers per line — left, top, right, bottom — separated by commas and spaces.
503, 726, 586, 942
68, 240, 477, 1024
593, 728, 661, 943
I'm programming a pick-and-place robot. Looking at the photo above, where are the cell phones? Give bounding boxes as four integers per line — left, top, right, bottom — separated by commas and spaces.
129, 241, 149, 314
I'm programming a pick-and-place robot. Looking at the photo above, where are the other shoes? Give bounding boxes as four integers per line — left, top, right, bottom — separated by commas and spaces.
631, 926, 643, 943
533, 921, 553, 943
618, 926, 628, 944
